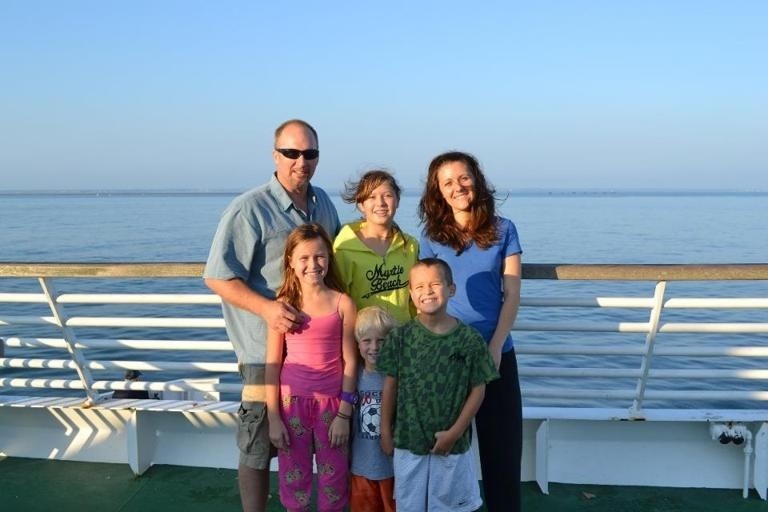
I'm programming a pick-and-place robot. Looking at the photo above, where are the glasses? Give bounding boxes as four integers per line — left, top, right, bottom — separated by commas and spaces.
273, 147, 320, 160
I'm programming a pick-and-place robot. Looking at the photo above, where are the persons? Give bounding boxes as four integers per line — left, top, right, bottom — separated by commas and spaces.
112, 370, 149, 399
